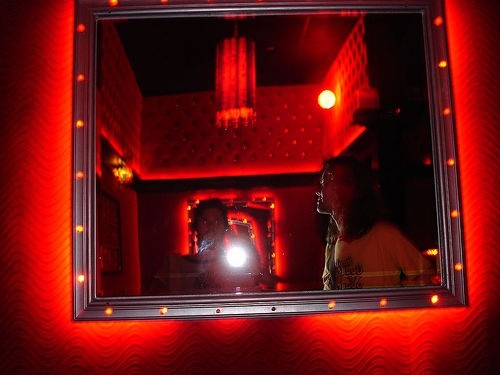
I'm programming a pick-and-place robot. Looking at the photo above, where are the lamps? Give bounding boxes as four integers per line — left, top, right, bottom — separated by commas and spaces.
213, 17, 258, 130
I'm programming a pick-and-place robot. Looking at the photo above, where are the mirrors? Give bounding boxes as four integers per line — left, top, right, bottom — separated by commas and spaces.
70, 0, 467, 321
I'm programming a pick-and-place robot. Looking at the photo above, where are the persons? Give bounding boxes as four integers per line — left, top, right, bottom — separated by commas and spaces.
316, 155, 434, 291
168, 198, 263, 294
